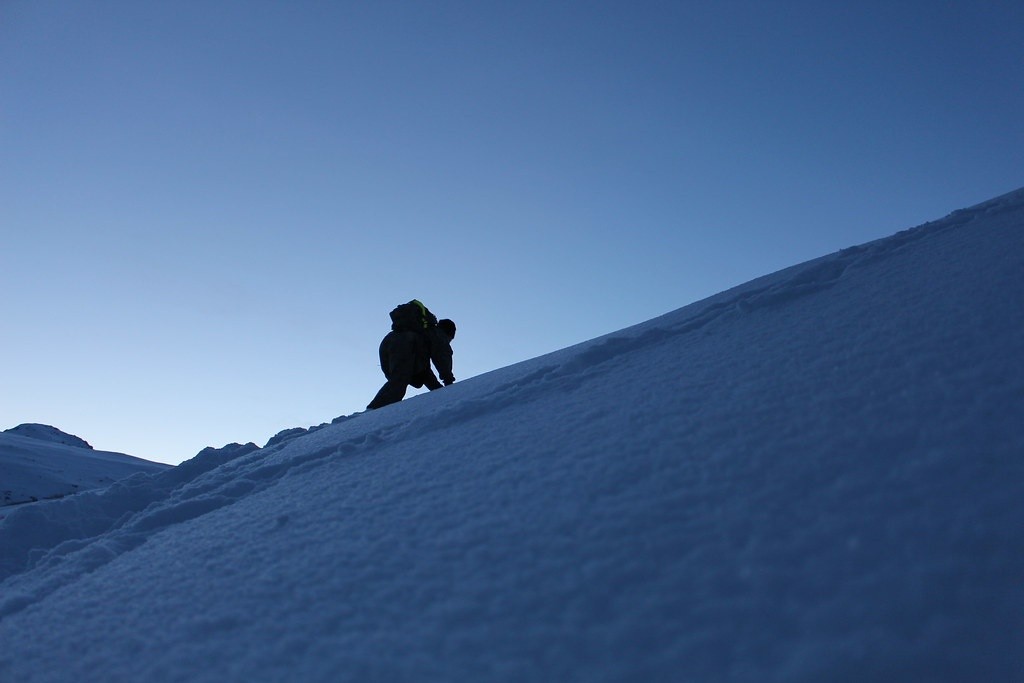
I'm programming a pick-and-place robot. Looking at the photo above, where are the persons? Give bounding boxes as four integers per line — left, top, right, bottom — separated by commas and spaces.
366, 318, 456, 410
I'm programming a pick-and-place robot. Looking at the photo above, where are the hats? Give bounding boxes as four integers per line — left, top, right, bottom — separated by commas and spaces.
437, 318, 456, 339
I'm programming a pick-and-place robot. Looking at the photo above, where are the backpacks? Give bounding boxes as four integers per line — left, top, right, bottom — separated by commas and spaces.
389, 298, 438, 331
